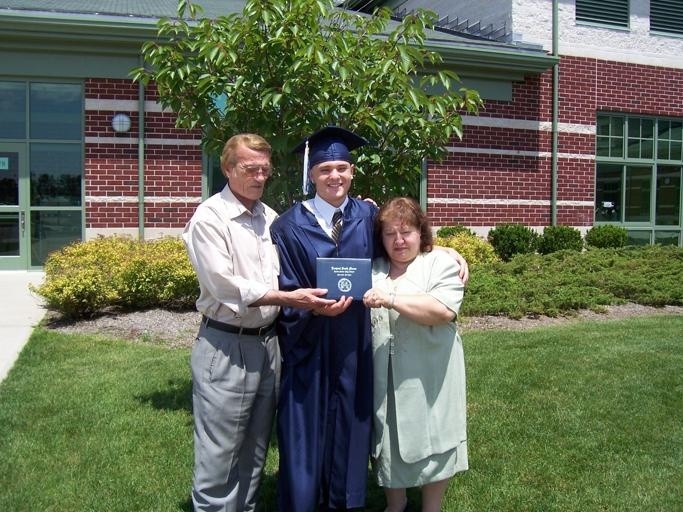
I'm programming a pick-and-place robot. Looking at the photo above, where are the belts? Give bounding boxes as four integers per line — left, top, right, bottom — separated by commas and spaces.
203, 314, 275, 335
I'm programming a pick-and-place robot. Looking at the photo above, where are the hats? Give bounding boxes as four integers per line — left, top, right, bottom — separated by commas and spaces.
292, 126, 368, 196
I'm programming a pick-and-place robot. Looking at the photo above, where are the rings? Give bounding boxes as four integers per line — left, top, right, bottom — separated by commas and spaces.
320, 307, 327, 314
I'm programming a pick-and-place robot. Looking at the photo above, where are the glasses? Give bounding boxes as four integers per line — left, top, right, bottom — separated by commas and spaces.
230, 162, 274, 177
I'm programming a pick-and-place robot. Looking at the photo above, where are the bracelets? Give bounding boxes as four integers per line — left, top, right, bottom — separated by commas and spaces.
387, 294, 395, 311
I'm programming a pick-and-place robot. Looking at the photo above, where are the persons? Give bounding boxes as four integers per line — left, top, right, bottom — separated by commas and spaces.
269, 127, 471, 512
364, 197, 472, 512
181, 132, 381, 512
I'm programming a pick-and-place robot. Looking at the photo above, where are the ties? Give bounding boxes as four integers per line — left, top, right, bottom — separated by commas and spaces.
332, 211, 343, 242
